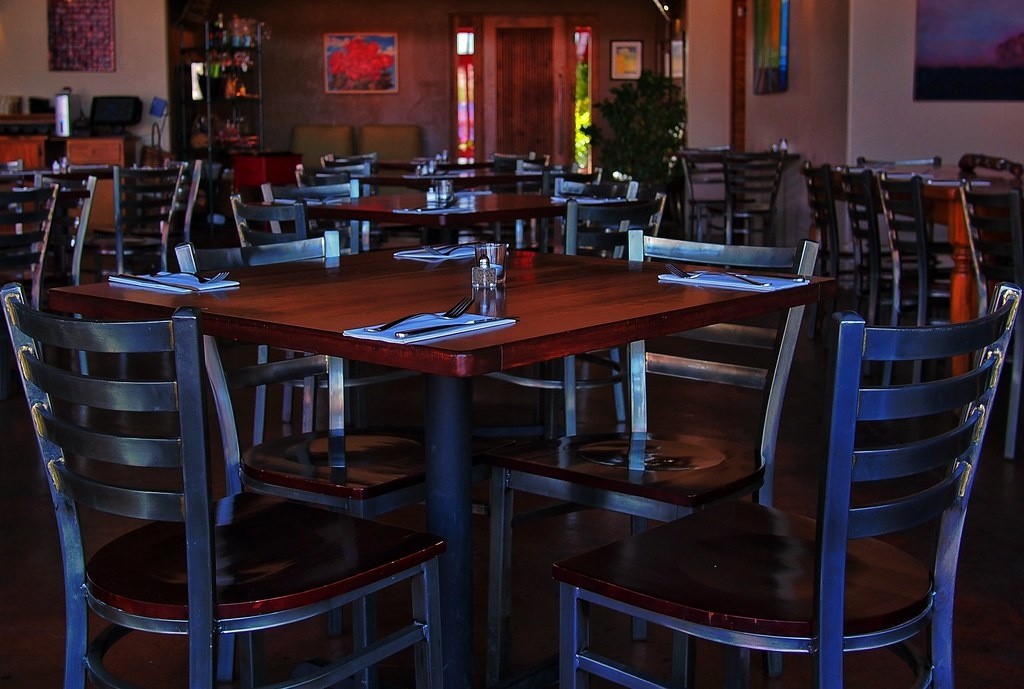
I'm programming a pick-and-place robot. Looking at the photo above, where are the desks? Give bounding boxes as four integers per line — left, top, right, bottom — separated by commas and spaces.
0, 142, 1024, 689
46, 247, 836, 689
232, 150, 301, 233
306, 194, 566, 245
884, 166, 1024, 375
377, 158, 497, 169
355, 171, 541, 193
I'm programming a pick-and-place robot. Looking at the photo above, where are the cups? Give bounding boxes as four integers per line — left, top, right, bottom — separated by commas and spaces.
438, 179, 453, 199
487, 285, 508, 316
471, 241, 509, 285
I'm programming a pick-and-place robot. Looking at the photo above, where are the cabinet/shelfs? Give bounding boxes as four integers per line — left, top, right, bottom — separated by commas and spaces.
174, 1, 264, 244
0, 134, 141, 237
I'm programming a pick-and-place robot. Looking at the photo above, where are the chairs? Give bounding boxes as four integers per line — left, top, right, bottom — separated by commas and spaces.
292, 124, 352, 169
360, 123, 421, 158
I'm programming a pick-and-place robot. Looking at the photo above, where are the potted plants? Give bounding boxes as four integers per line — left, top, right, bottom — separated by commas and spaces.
580, 69, 686, 216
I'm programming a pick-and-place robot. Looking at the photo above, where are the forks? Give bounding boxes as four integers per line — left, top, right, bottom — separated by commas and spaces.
421, 241, 483, 255
415, 196, 458, 211
395, 241, 480, 257
664, 263, 772, 287
363, 296, 475, 333
150, 272, 230, 284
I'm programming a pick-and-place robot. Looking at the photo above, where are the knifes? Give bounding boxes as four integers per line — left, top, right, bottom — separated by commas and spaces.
117, 273, 201, 292
394, 316, 520, 340
687, 272, 806, 283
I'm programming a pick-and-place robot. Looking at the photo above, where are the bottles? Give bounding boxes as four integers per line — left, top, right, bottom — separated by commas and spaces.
471, 287, 496, 317
554, 178, 563, 197
469, 255, 496, 287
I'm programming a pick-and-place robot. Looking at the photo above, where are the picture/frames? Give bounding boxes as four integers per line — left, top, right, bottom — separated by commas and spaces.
609, 39, 644, 81
323, 31, 399, 94
